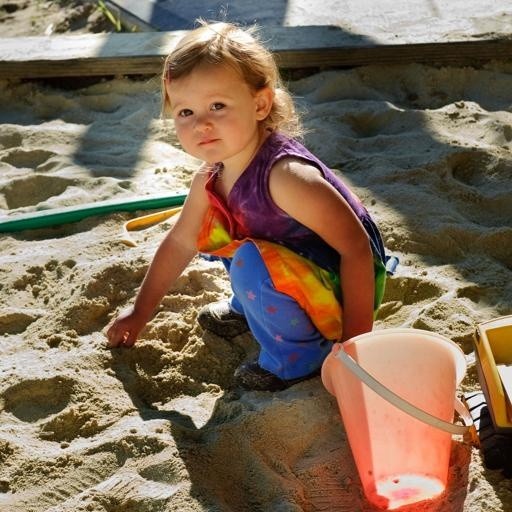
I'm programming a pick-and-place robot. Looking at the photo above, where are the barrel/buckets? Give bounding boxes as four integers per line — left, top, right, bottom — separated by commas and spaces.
320, 326, 476, 510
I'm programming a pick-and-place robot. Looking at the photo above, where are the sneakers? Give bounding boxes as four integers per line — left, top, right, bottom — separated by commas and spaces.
197, 297, 249, 340
233, 355, 322, 392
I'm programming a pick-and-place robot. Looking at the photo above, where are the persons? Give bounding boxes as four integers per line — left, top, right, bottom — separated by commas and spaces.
104, 20, 387, 394
